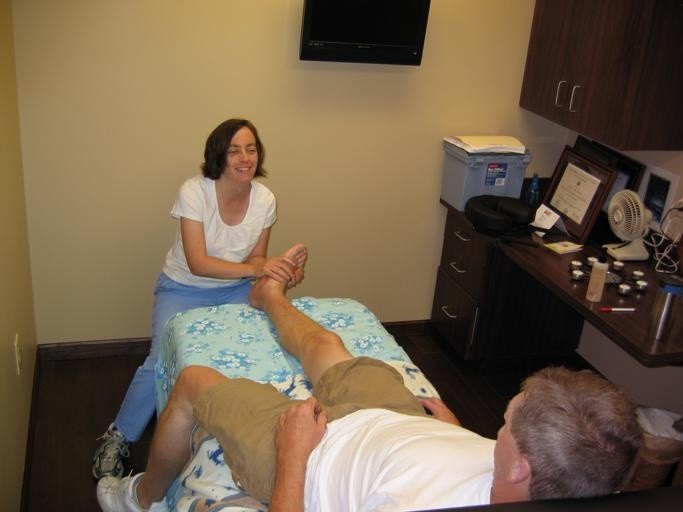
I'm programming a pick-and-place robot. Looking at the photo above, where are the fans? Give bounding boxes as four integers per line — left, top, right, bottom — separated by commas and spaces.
603, 189, 655, 261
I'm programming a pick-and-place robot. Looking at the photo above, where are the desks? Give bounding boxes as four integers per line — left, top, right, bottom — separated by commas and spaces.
429, 197, 682, 412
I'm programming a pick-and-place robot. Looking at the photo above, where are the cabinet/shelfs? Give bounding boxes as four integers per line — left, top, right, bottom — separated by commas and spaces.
518, 1, 683, 152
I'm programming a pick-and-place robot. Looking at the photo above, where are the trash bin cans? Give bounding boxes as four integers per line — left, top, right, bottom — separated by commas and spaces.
621, 405, 683, 493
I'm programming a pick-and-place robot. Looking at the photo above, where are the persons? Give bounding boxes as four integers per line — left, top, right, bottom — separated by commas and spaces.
91, 237, 643, 512
88, 116, 308, 484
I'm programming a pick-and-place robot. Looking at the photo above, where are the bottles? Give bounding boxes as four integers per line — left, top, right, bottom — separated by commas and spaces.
526, 173, 540, 208
584, 257, 610, 303
646, 275, 683, 342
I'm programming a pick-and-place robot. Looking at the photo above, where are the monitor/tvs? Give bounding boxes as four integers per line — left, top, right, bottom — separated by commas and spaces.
297, 0, 431, 67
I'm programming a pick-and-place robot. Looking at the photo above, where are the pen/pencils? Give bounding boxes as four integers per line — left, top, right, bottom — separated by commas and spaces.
597, 307, 640, 312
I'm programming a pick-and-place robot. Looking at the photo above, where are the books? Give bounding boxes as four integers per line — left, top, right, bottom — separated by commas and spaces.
437, 134, 525, 156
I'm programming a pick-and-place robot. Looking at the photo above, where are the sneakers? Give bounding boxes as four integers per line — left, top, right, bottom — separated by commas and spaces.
97, 473, 168, 512
93, 423, 128, 479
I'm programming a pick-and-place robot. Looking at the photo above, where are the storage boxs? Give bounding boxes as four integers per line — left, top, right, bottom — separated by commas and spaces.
441, 140, 530, 212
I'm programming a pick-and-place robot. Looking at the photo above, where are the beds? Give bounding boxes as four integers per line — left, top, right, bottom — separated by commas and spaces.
156, 297, 680, 511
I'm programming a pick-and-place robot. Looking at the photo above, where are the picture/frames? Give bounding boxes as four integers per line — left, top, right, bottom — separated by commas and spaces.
535, 136, 647, 245
